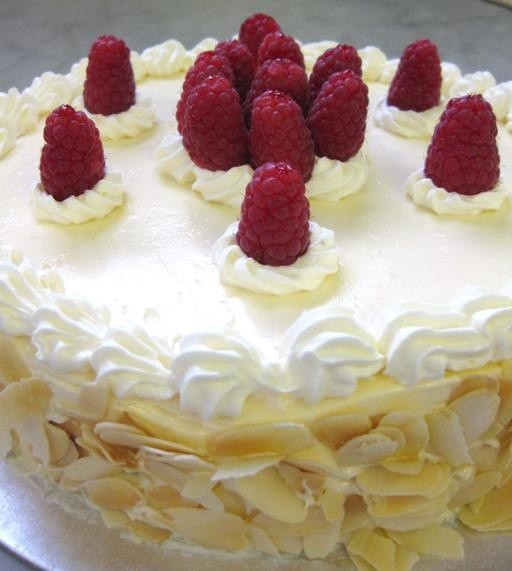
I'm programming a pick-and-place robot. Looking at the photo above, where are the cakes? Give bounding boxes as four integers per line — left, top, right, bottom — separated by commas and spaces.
0, 13, 512, 571
